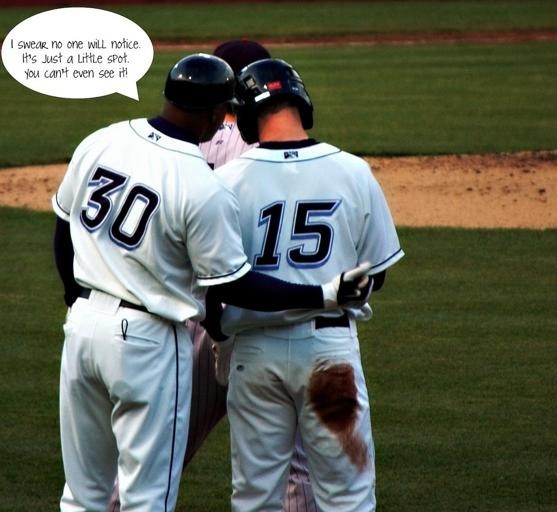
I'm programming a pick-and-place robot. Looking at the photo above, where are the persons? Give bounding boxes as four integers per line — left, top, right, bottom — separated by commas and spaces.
205, 59, 405, 512
49, 52, 375, 511
110, 40, 319, 510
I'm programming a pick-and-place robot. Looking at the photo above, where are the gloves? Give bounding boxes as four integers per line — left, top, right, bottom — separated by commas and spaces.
210, 336, 236, 386
319, 263, 376, 311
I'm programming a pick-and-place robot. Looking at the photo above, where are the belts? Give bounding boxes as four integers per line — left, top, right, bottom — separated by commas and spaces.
79, 289, 147, 312
314, 313, 350, 331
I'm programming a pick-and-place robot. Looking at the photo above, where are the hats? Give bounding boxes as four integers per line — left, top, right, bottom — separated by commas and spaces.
213, 40, 272, 74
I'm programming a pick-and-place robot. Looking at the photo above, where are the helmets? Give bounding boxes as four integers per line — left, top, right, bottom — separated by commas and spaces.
237, 57, 313, 144
164, 52, 238, 114
